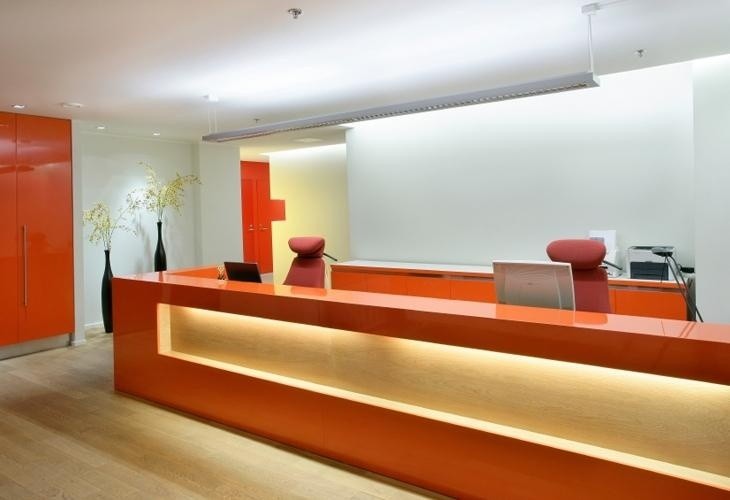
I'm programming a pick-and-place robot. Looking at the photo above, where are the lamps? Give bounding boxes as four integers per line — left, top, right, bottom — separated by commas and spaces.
651, 246, 702, 323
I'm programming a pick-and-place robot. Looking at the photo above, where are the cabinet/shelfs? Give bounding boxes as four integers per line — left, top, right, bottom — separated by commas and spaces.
0, 111, 75, 361
240, 160, 274, 285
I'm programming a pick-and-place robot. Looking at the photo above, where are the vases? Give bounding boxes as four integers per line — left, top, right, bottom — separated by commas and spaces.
101, 249, 113, 333
153, 221, 167, 272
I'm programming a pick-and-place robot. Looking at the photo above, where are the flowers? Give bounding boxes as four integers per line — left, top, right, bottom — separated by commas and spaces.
82, 202, 136, 251
127, 160, 202, 218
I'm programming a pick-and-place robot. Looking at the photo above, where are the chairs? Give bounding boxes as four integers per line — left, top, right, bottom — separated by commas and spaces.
281, 236, 327, 290
546, 239, 611, 314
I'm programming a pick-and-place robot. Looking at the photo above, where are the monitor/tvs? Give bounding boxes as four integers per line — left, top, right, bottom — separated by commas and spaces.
491, 260, 576, 312
224, 262, 262, 283
